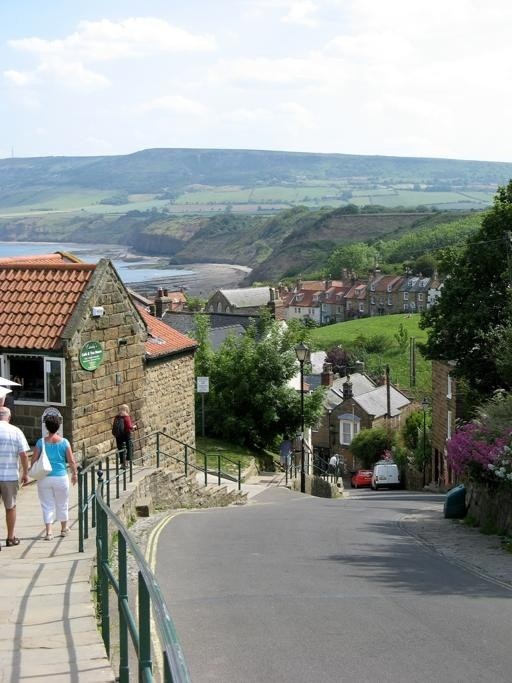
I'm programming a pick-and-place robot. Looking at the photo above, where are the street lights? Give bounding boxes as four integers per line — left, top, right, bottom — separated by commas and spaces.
327, 408, 333, 446
292, 338, 310, 493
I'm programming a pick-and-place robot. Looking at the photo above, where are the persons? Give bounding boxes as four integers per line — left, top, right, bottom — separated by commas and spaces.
0, 405, 29, 547
113, 404, 137, 469
328, 453, 338, 474
279, 434, 294, 472
31, 411, 78, 541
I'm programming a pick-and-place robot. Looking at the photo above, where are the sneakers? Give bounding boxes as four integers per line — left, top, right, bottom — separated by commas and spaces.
44, 533, 54, 541
6, 536, 20, 546
61, 527, 70, 537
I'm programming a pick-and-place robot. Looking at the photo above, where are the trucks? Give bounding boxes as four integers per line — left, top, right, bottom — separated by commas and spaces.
371, 462, 401, 489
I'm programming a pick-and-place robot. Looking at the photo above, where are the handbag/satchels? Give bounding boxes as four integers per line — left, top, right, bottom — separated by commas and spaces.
27, 437, 53, 481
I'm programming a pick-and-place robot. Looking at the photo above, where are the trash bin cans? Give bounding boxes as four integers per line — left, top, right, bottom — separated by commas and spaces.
444, 483, 467, 519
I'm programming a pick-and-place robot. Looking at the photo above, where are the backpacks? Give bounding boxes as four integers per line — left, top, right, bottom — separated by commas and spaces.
112, 415, 124, 437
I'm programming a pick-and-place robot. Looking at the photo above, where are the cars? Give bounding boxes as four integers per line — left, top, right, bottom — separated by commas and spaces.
352, 468, 374, 488
